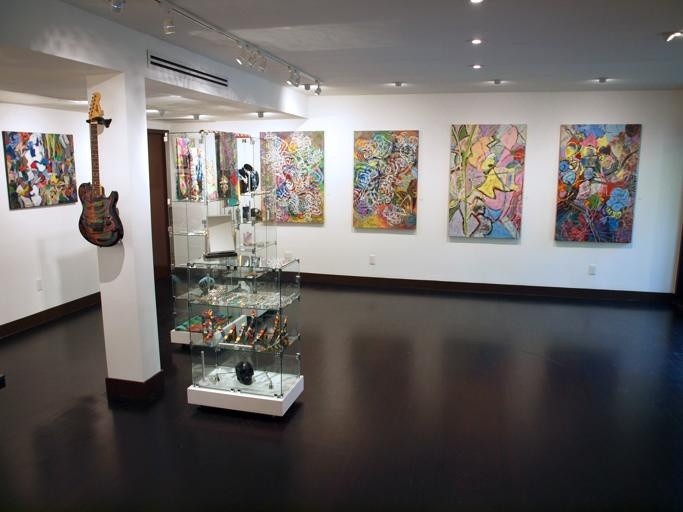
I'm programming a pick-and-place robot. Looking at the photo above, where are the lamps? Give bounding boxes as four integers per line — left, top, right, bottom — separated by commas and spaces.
112, 0, 321, 95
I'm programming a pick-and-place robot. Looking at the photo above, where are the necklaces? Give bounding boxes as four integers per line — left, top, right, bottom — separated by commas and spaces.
240, 167, 256, 177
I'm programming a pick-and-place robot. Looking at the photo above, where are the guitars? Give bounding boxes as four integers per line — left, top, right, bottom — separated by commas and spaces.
79, 90, 123, 247
